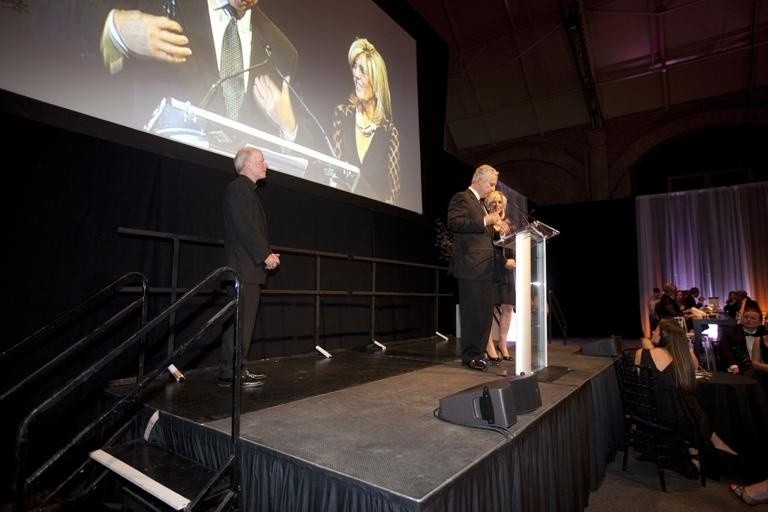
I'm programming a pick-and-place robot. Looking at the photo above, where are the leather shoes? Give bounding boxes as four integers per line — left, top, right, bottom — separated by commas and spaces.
467, 345, 513, 369
219, 371, 266, 389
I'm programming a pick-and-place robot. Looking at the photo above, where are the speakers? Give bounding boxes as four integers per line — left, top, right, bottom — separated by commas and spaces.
580, 335, 624, 358
438, 371, 542, 430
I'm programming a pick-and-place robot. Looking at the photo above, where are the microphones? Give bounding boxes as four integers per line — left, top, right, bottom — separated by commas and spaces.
507, 200, 536, 225
196, 26, 338, 160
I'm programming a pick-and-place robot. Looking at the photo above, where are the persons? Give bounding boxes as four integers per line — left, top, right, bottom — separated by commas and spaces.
216, 146, 282, 389
332, 35, 402, 209
633, 279, 768, 507
483, 190, 516, 362
446, 163, 510, 371
73, 0, 310, 148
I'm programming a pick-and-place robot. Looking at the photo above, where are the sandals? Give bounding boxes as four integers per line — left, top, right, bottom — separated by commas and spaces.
730, 484, 761, 506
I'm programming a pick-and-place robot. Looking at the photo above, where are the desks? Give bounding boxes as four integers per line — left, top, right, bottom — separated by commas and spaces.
697, 369, 768, 482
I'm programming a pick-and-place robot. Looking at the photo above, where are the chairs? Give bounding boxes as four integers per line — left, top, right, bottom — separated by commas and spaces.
613, 359, 707, 492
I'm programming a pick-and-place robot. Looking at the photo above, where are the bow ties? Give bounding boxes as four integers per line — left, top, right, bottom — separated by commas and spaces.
743, 330, 760, 337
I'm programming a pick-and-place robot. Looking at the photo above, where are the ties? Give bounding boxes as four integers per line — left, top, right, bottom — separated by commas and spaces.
219, 8, 246, 120
